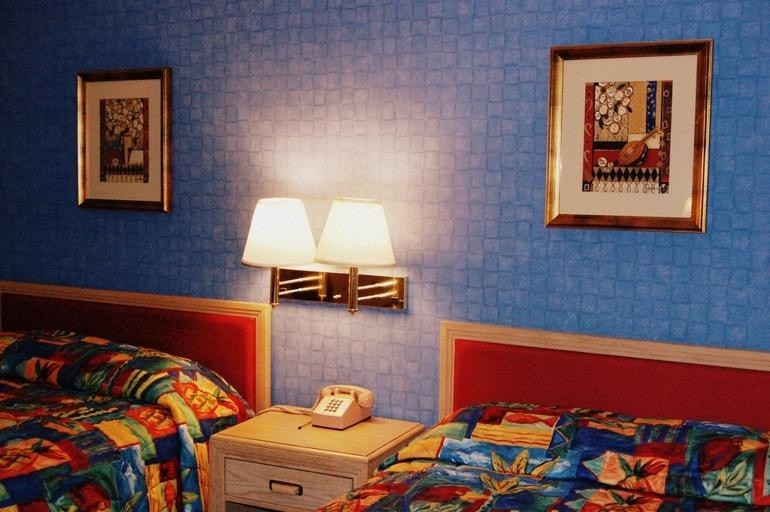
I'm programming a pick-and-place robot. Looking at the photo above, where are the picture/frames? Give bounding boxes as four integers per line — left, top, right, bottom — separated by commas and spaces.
76, 66, 173, 215
545, 39, 715, 235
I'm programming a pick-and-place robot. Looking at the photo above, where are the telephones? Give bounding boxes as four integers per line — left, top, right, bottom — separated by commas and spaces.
312, 385, 374, 429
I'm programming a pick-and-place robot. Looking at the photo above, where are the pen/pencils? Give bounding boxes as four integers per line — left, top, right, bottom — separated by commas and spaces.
298, 421, 312, 429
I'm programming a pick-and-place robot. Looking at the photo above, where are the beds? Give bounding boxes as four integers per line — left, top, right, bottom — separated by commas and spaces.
314, 318, 770, 512
1, 277, 272, 511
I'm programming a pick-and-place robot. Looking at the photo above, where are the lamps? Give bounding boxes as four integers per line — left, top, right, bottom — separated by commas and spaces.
239, 195, 410, 311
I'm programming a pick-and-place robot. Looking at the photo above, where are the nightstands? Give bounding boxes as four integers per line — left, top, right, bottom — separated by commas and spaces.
209, 402, 428, 509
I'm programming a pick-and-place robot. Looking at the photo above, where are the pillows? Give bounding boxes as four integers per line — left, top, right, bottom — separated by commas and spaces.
376, 401, 770, 506
